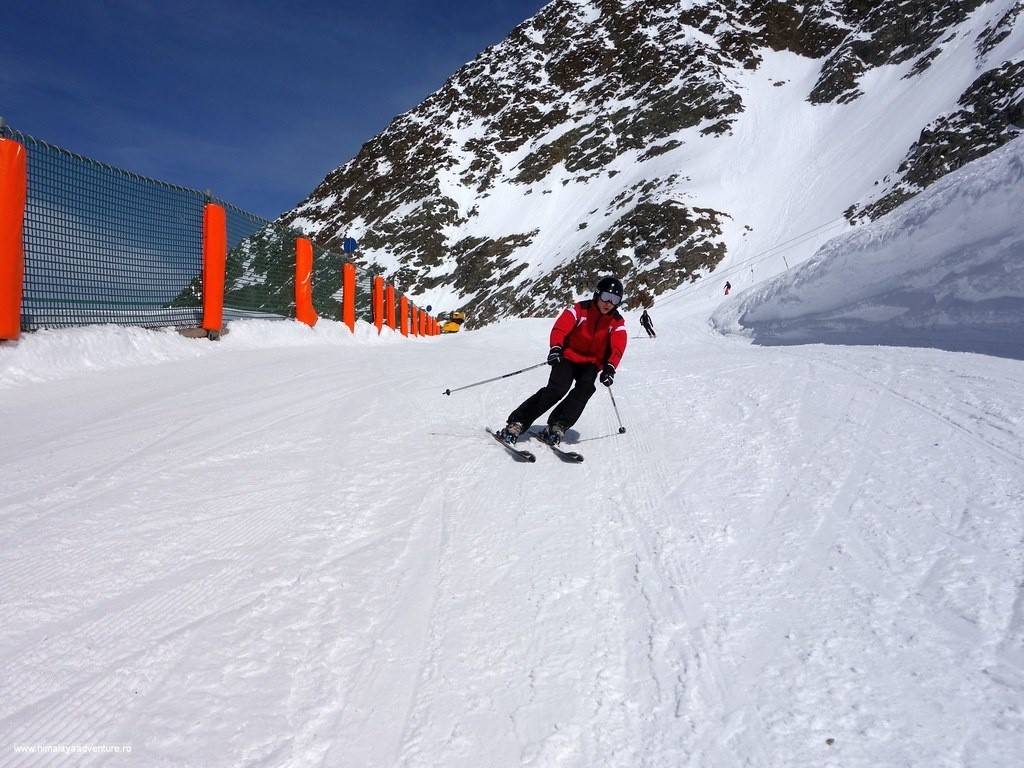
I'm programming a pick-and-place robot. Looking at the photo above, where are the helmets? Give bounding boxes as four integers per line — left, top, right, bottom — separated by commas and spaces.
593, 277, 623, 316
643, 310, 647, 316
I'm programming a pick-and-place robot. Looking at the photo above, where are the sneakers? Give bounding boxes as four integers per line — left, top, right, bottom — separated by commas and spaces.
505, 422, 524, 439
548, 424, 566, 439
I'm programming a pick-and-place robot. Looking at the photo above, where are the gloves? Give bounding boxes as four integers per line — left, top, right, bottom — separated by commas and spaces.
650, 322, 652, 324
600, 365, 616, 386
547, 345, 562, 365
641, 322, 642, 325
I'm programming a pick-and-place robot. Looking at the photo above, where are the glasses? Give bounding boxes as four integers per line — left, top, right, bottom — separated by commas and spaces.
599, 291, 620, 305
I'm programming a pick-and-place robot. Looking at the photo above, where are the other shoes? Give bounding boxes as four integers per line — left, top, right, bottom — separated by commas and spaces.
649, 334, 657, 339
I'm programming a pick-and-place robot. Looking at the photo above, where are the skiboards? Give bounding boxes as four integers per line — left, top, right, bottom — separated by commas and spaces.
650, 335, 656, 339
485, 426, 584, 463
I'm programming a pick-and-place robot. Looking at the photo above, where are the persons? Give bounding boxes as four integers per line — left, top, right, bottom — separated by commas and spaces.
639, 310, 656, 339
724, 281, 731, 295
505, 278, 627, 444
